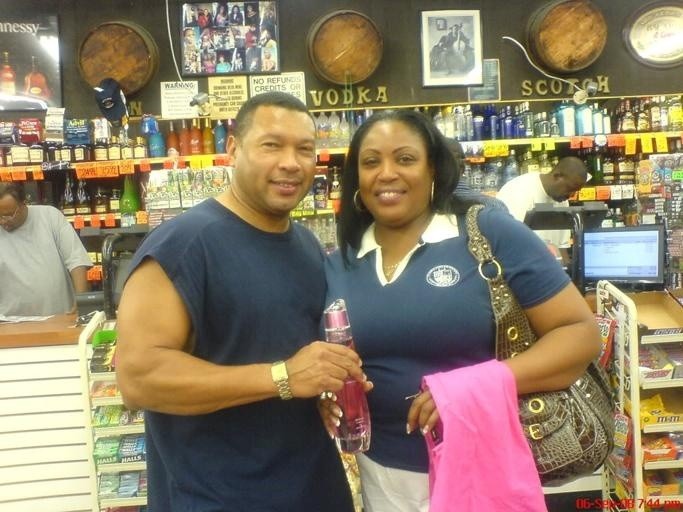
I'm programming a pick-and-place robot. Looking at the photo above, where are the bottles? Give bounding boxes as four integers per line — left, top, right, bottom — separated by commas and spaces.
109, 117, 233, 159
24, 55, 53, 101
310, 95, 683, 200
0, 51, 18, 95
322, 298, 374, 455
62, 170, 141, 228
293, 217, 337, 254
601, 204, 637, 228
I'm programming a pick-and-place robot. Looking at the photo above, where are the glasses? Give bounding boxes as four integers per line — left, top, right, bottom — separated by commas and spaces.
0, 207, 18, 220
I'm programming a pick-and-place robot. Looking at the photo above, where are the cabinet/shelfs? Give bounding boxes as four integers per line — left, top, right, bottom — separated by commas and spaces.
0, 92, 682, 291
595, 277, 683, 512
78, 313, 151, 512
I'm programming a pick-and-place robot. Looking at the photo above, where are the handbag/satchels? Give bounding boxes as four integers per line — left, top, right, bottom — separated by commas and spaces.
489, 274, 617, 486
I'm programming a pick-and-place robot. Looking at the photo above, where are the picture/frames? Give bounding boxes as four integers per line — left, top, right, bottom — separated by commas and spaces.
0, 10, 64, 112
421, 9, 484, 89
177, 0, 282, 78
468, 58, 501, 103
620, 0, 683, 71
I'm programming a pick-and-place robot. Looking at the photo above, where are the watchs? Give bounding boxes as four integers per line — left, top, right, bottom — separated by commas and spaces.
271, 359, 292, 400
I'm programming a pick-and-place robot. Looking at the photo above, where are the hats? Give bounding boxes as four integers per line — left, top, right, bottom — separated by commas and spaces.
94, 79, 130, 128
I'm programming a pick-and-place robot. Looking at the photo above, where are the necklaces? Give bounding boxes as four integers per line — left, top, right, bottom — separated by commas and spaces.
383, 262, 401, 278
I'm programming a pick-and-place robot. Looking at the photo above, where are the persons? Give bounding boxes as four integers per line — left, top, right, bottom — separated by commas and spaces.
317, 110, 602, 512
115, 92, 367, 512
0, 182, 94, 316
496, 155, 587, 266
438, 25, 469, 75
181, 3, 278, 73
444, 137, 508, 213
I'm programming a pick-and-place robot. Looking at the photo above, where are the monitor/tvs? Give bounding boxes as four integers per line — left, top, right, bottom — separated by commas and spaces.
582, 224, 665, 286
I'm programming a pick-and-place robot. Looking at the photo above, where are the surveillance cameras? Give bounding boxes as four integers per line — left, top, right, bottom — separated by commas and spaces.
587, 81, 598, 96
188, 91, 208, 107
